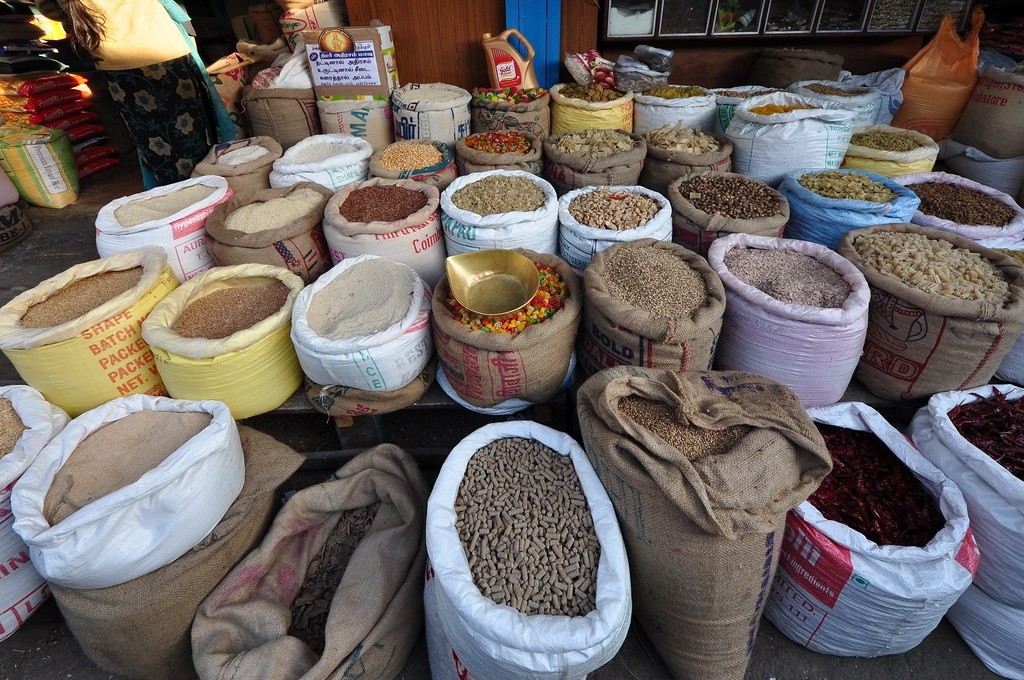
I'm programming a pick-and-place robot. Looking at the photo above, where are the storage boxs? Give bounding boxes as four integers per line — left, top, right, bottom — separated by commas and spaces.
301, 25, 401, 99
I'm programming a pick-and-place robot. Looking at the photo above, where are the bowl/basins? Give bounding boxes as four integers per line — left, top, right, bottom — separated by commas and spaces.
444, 248, 541, 319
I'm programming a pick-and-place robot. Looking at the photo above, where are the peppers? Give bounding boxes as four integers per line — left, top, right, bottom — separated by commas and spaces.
803, 421, 944, 548
947, 385, 1024, 484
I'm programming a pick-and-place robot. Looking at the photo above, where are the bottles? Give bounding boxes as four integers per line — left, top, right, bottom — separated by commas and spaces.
482, 29, 539, 91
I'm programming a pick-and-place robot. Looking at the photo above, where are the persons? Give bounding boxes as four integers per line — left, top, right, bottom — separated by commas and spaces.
35, 0, 237, 191
